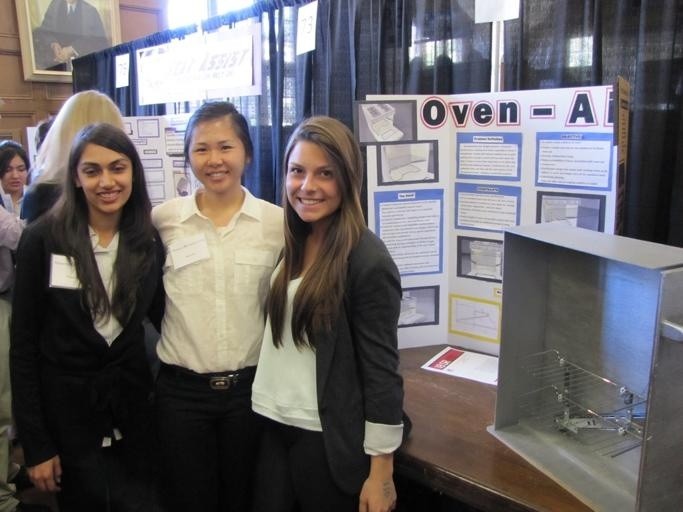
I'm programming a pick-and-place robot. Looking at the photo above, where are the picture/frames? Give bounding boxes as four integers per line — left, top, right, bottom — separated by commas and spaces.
13, 1, 121, 84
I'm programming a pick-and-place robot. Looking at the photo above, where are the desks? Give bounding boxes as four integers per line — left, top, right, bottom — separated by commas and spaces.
391, 340, 590, 510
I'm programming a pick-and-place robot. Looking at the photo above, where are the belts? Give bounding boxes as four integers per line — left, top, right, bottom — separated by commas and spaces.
159, 364, 257, 392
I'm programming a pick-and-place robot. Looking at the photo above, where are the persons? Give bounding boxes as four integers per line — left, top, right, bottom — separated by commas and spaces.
150, 101, 287, 510
11, 124, 165, 512
32, 0, 108, 72
0, 139, 30, 215
250, 115, 412, 512
20, 90, 124, 223
0, 206, 54, 512
